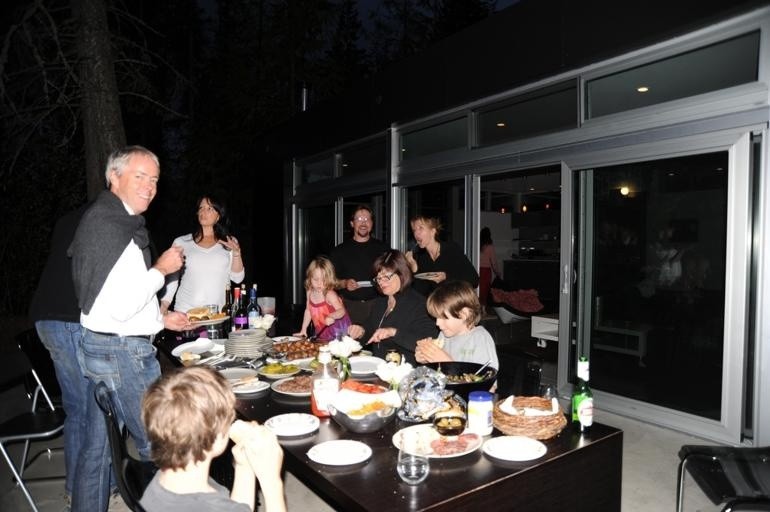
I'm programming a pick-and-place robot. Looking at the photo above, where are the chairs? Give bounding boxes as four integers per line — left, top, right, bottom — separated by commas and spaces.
0, 324, 65, 512
487, 278, 547, 339
93, 380, 260, 512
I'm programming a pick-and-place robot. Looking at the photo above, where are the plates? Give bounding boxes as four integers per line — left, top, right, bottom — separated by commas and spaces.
171, 342, 200, 357
217, 362, 311, 398
224, 329, 274, 357
415, 272, 439, 279
264, 413, 320, 437
266, 355, 340, 372
391, 425, 480, 459
349, 356, 385, 375
188, 315, 231, 325
356, 281, 375, 288
272, 336, 299, 342
485, 435, 549, 465
307, 440, 371, 467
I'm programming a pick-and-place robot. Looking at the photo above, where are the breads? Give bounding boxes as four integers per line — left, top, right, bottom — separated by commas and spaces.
186, 307, 227, 322
228, 419, 254, 449
417, 337, 444, 349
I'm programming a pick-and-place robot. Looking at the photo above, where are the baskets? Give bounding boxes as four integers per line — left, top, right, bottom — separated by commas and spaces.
493, 396, 567, 444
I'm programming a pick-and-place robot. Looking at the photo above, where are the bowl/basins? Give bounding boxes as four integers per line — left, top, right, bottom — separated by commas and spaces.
328, 394, 396, 435
419, 362, 498, 402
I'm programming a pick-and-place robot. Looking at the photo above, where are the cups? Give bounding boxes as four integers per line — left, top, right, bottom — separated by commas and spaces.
396, 445, 429, 483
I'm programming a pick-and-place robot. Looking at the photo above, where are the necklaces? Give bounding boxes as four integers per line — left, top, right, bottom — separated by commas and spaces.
386, 298, 397, 312
428, 242, 440, 260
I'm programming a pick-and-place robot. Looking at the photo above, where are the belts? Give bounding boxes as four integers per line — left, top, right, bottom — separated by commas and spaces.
88, 329, 151, 341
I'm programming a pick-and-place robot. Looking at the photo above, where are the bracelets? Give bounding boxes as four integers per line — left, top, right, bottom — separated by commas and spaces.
232, 253, 241, 258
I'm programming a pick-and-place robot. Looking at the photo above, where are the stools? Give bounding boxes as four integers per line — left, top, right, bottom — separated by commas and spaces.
674, 443, 770, 511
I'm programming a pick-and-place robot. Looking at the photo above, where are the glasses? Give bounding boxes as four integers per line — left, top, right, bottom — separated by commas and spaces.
372, 272, 396, 283
353, 217, 372, 221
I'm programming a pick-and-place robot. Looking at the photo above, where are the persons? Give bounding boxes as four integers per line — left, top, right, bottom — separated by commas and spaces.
407, 210, 480, 297
290, 255, 355, 342
26, 187, 121, 508
139, 364, 294, 512
477, 227, 504, 315
654, 236, 683, 288
69, 142, 189, 512
159, 196, 248, 327
347, 250, 443, 367
412, 278, 500, 395
330, 206, 398, 325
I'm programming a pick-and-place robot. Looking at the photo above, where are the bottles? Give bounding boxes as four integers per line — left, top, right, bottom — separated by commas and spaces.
467, 391, 494, 436
385, 349, 400, 364
206, 304, 220, 339
310, 345, 342, 418
568, 355, 594, 435
221, 283, 263, 338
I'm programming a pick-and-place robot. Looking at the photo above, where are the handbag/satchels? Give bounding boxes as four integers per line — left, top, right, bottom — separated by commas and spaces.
154, 327, 198, 368
